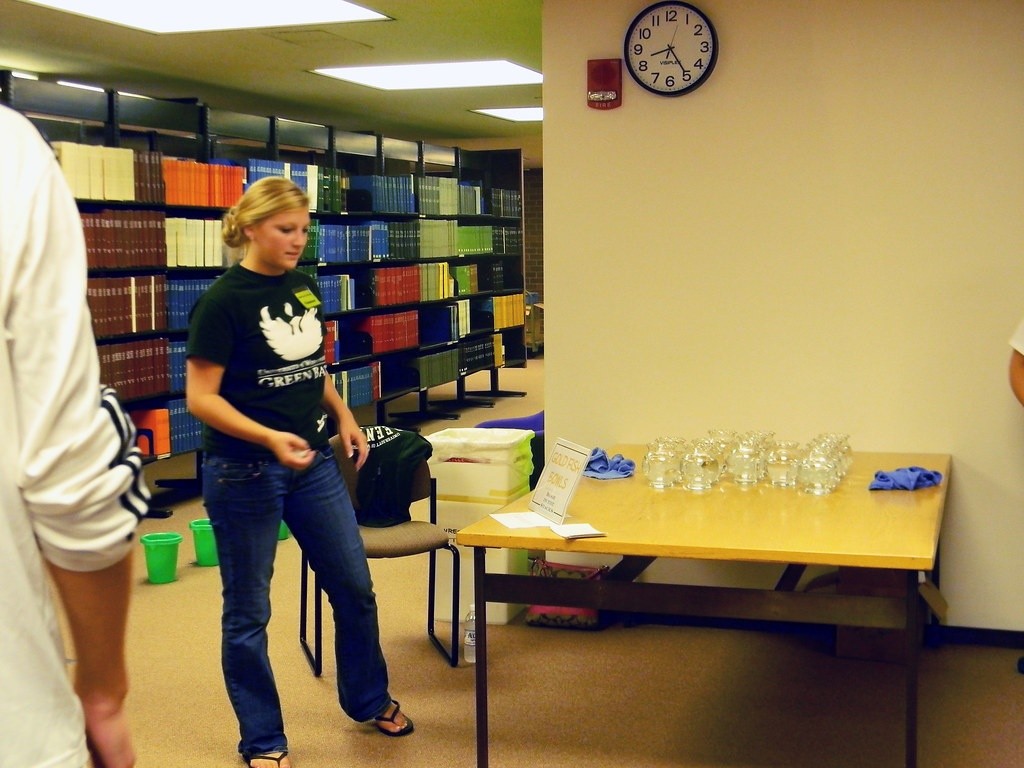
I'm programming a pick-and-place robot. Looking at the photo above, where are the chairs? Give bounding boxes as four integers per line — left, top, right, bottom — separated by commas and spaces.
300, 425, 461, 678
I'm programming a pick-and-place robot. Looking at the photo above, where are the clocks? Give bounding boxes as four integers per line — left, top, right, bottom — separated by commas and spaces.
624, 0, 719, 98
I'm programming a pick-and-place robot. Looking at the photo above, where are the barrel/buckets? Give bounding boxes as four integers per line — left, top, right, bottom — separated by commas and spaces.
141, 532, 184, 584
188, 519, 221, 566
278, 519, 290, 539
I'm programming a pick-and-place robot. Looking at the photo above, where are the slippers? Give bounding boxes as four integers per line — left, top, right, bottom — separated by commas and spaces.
374, 699, 413, 736
247, 750, 291, 768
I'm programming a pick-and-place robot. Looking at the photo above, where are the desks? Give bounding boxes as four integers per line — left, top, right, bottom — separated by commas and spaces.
459, 443, 954, 768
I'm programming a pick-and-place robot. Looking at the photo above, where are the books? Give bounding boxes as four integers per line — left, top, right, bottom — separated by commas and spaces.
49, 137, 528, 472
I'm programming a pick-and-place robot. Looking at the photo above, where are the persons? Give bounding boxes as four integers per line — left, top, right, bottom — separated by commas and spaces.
184, 175, 415, 768
1008, 315, 1024, 676
0, 105, 151, 767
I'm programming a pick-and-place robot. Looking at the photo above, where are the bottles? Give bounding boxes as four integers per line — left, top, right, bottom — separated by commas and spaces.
464, 604, 476, 663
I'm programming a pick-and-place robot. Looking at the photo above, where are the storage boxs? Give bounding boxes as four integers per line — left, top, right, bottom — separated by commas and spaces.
422, 427, 536, 505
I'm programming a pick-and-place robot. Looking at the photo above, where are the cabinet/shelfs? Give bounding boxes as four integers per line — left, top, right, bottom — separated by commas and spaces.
0, 69, 528, 520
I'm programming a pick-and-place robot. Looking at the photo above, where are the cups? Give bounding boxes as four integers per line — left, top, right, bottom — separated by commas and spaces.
644, 430, 847, 494
